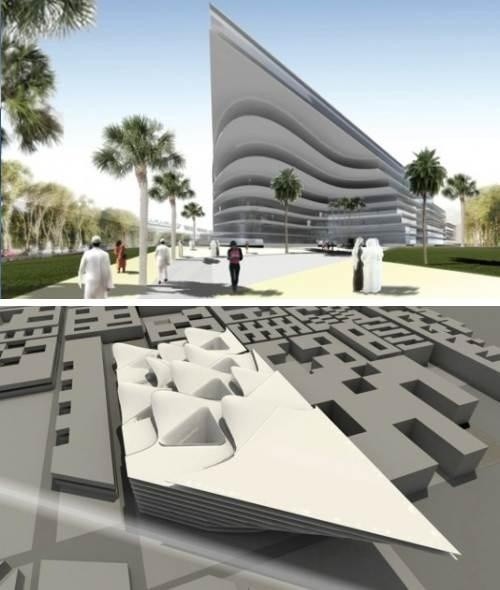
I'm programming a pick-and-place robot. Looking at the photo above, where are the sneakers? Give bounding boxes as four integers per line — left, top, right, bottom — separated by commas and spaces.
117, 270, 125, 273
158, 278, 168, 284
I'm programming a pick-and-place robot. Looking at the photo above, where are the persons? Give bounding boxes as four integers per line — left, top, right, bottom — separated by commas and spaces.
76, 233, 116, 299
226, 240, 244, 292
112, 237, 128, 273
153, 237, 172, 284
349, 237, 386, 294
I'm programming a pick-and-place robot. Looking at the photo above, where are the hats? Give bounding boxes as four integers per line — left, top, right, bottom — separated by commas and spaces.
92, 235, 101, 243
160, 239, 165, 242
